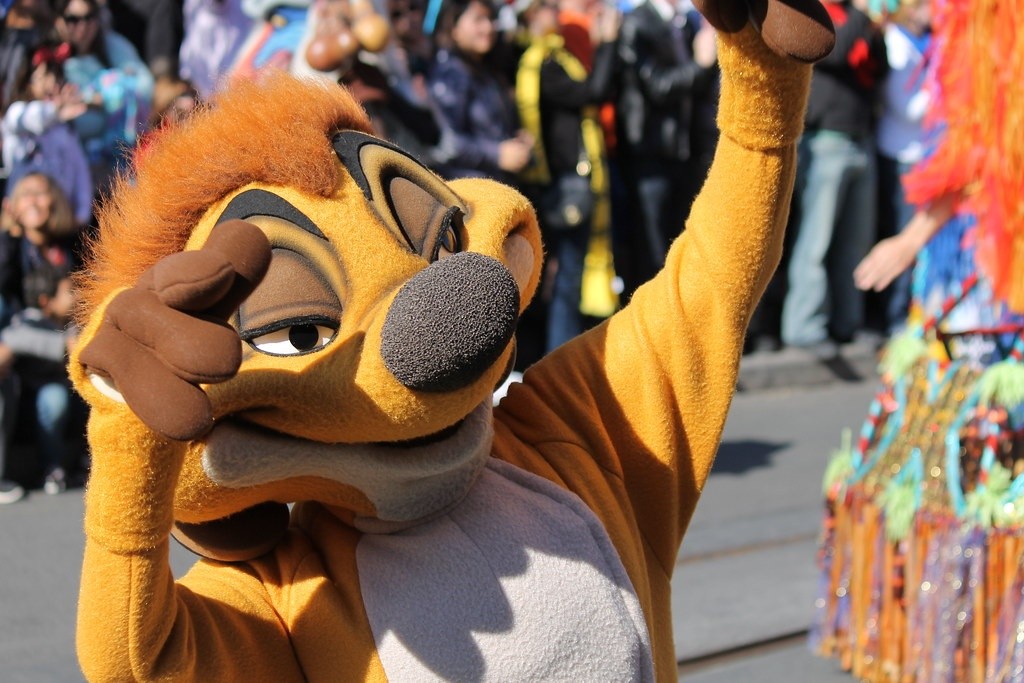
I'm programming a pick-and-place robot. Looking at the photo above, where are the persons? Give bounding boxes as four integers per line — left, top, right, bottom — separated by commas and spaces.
811, 1, 1024, 683
0, 0, 937, 501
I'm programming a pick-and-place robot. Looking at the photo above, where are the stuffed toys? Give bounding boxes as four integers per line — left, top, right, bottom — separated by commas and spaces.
69, 0, 834, 683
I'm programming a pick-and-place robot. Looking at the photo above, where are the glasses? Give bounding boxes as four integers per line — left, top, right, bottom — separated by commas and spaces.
61, 12, 98, 25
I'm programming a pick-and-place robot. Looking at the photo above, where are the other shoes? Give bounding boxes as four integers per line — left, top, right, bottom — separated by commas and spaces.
44, 468, 65, 496
806, 340, 839, 363
829, 329, 885, 353
0, 476, 25, 505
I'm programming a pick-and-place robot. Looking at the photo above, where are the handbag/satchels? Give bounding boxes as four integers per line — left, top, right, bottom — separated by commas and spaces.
543, 169, 591, 229
